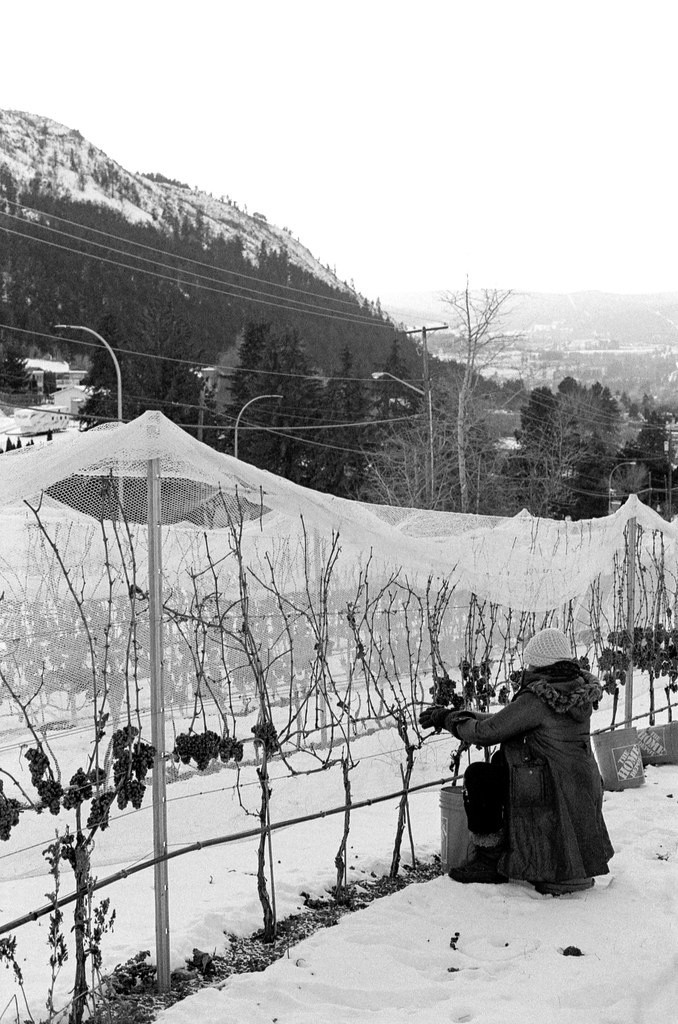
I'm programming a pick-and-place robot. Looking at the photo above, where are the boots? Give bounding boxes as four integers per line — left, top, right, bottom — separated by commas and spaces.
451, 832, 507, 885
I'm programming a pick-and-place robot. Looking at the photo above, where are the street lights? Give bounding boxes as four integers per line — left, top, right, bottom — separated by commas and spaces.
372, 369, 435, 510
234, 394, 284, 460
607, 460, 637, 516
56, 323, 126, 524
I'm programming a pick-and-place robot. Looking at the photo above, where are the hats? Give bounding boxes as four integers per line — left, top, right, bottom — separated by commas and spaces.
522, 629, 572, 668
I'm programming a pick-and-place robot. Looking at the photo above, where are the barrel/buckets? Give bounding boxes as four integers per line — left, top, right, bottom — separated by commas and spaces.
590, 727, 647, 789
640, 717, 678, 764
439, 783, 480, 873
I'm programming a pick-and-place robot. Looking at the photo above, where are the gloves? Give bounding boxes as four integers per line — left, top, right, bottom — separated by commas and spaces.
419, 705, 451, 730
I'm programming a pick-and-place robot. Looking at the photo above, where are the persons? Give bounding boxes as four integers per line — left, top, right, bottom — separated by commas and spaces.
415, 629, 616, 895
